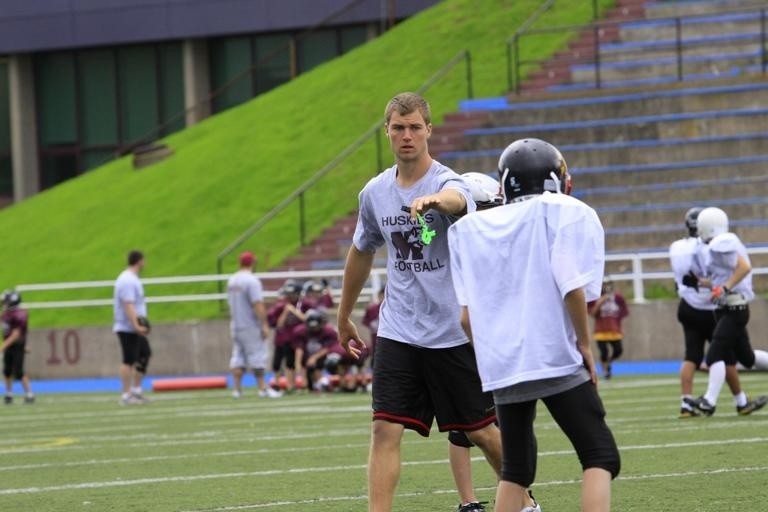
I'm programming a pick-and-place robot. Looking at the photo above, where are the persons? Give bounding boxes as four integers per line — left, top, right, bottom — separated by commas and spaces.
267, 277, 385, 398
226, 251, 282, 397
447, 171, 542, 512
0, 292, 35, 404
668, 206, 768, 419
586, 276, 629, 379
337, 93, 543, 512
683, 206, 768, 418
448, 139, 620, 512
112, 250, 156, 406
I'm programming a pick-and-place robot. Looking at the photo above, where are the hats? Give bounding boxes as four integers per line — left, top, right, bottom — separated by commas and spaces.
239, 251, 257, 267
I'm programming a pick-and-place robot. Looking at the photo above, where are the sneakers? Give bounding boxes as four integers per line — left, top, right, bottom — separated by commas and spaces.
678, 393, 717, 418
603, 362, 615, 380
118, 385, 155, 407
230, 387, 243, 401
257, 368, 374, 400
4, 393, 36, 405
736, 394, 768, 416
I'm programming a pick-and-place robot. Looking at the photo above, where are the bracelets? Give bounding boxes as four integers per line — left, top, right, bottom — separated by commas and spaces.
723, 285, 729, 294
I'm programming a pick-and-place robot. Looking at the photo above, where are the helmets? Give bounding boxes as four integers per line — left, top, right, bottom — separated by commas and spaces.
275, 277, 325, 329
1, 290, 22, 309
497, 137, 572, 205
684, 205, 730, 243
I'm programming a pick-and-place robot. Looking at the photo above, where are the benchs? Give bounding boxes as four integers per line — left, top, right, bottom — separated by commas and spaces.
309, 82, 768, 280
501, 4, 768, 95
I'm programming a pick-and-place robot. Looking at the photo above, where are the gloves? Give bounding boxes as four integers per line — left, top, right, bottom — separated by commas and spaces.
709, 285, 728, 306
682, 270, 699, 292
